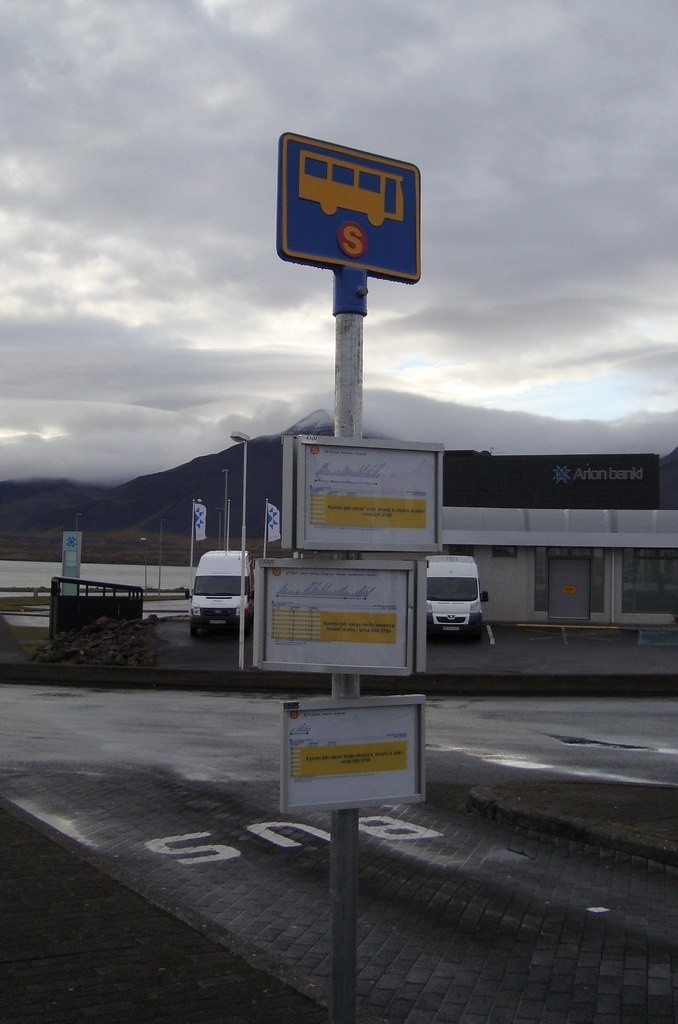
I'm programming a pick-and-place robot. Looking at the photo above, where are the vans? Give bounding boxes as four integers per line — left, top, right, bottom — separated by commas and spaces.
426, 554, 488, 644
186, 550, 255, 639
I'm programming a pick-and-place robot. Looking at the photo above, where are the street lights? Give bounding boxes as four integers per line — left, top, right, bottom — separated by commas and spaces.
222, 469, 229, 550
230, 431, 250, 669
75, 510, 82, 578
140, 537, 147, 597
158, 518, 166, 596
